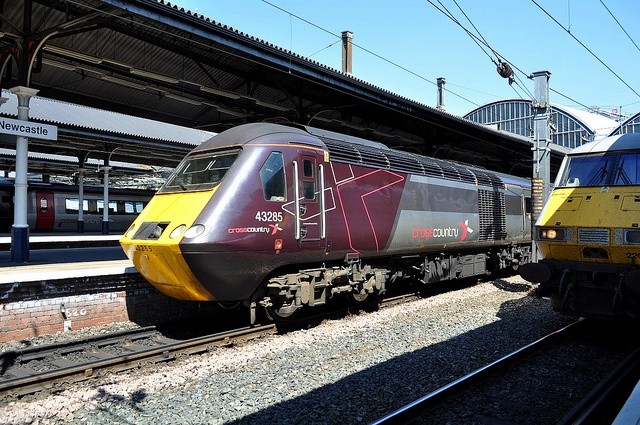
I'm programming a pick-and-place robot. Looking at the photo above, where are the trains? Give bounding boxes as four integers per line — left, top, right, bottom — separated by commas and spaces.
118, 123, 531, 327
533, 133, 640, 320
0, 176, 157, 233
